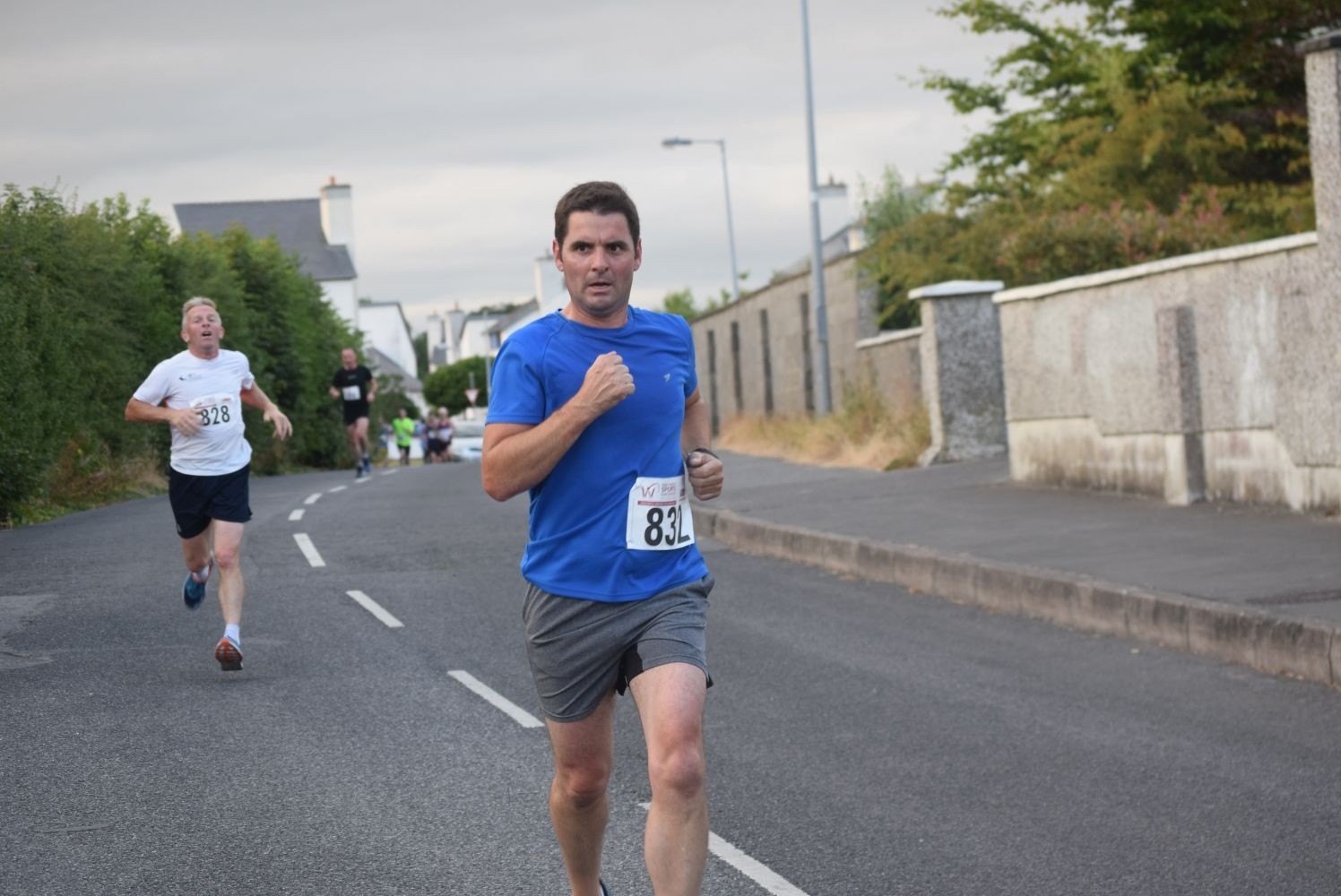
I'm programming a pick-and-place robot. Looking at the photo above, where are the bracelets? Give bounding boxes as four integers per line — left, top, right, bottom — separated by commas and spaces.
686, 448, 718, 461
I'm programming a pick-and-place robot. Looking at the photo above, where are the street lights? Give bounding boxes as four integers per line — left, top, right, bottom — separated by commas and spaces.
660, 137, 741, 301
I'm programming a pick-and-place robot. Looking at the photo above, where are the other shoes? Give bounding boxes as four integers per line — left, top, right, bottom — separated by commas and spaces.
357, 466, 363, 477
363, 458, 372, 472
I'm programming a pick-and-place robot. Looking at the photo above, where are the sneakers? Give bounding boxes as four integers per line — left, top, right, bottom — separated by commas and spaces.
182, 552, 214, 610
215, 636, 244, 671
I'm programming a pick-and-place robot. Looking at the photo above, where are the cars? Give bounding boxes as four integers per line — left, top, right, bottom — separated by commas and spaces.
447, 421, 489, 463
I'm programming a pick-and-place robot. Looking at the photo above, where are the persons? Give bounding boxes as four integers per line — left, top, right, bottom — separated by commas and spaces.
376, 406, 456, 467
481, 181, 725, 896
328, 346, 377, 477
125, 296, 294, 670
